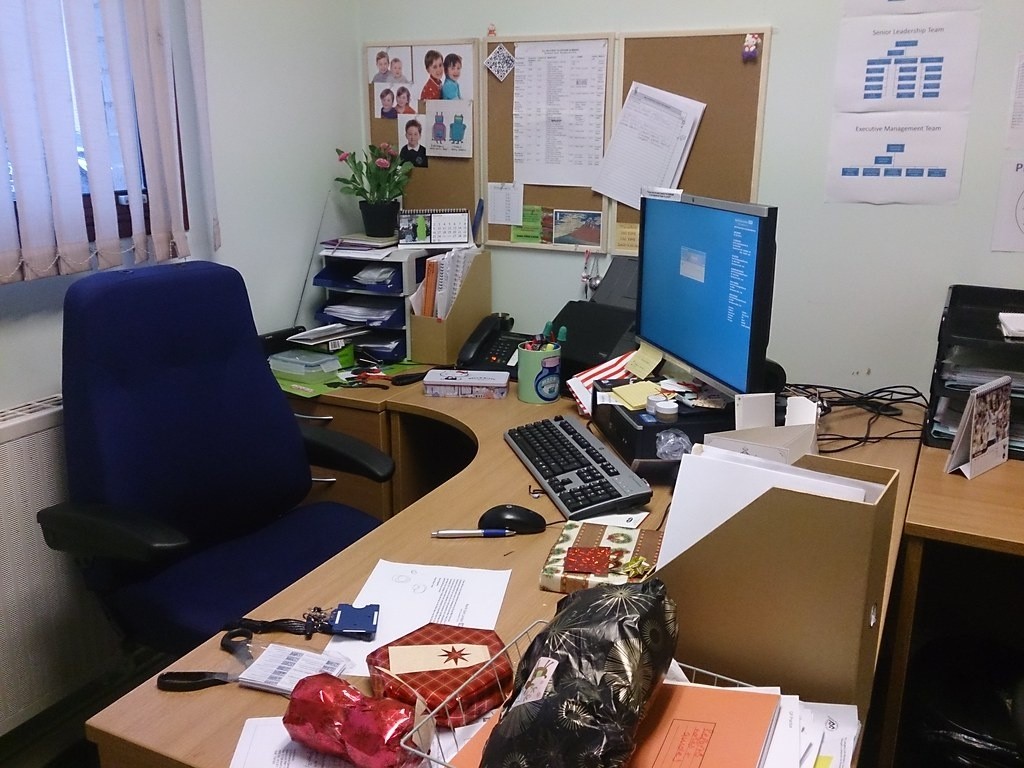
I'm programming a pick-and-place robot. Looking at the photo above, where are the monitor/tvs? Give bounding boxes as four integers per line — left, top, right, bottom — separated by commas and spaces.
634, 185, 787, 416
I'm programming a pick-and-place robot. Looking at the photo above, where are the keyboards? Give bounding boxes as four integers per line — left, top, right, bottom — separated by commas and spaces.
503, 414, 653, 522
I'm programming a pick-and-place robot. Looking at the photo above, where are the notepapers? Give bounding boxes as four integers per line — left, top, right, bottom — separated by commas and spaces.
611, 381, 673, 408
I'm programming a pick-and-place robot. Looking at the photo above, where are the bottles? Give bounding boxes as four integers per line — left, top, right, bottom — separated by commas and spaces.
646, 394, 679, 423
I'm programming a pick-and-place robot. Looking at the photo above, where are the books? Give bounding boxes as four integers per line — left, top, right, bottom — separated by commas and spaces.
424, 245, 482, 320
287, 322, 371, 345
932, 345, 1024, 453
319, 229, 399, 251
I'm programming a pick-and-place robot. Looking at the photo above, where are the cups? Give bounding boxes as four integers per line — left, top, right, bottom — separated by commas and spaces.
517, 340, 563, 405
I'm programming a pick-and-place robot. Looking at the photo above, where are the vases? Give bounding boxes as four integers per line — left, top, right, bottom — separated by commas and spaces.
359, 200, 401, 238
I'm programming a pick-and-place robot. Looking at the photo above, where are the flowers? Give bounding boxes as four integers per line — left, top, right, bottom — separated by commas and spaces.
335, 144, 414, 203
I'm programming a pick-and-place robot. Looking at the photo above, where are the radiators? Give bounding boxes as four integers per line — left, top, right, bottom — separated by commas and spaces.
0, 393, 130, 737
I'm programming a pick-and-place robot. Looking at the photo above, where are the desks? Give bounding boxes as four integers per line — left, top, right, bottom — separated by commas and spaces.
86, 362, 1024, 768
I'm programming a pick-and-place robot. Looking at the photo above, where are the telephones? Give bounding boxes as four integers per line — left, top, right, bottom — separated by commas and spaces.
455, 312, 536, 382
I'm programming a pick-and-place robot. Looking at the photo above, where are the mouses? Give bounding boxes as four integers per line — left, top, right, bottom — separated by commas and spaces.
478, 504, 546, 535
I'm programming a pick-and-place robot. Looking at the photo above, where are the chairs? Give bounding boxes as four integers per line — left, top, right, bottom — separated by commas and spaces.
36, 259, 393, 658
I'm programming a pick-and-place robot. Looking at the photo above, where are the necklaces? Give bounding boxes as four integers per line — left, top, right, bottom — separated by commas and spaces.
429, 77, 442, 87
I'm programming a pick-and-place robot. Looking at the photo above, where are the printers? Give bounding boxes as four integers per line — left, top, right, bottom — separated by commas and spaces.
543, 255, 639, 397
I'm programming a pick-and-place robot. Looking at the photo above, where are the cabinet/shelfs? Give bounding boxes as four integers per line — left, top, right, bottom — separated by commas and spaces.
319, 245, 440, 365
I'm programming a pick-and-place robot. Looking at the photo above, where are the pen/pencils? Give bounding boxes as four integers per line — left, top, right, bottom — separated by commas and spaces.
524, 331, 559, 351
432, 529, 518, 537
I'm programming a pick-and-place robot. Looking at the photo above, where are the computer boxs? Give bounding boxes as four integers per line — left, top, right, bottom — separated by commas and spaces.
590, 376, 787, 467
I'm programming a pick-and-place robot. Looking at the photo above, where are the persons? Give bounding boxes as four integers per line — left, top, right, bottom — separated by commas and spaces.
400, 219, 430, 242
371, 51, 462, 168
972, 384, 1009, 456
